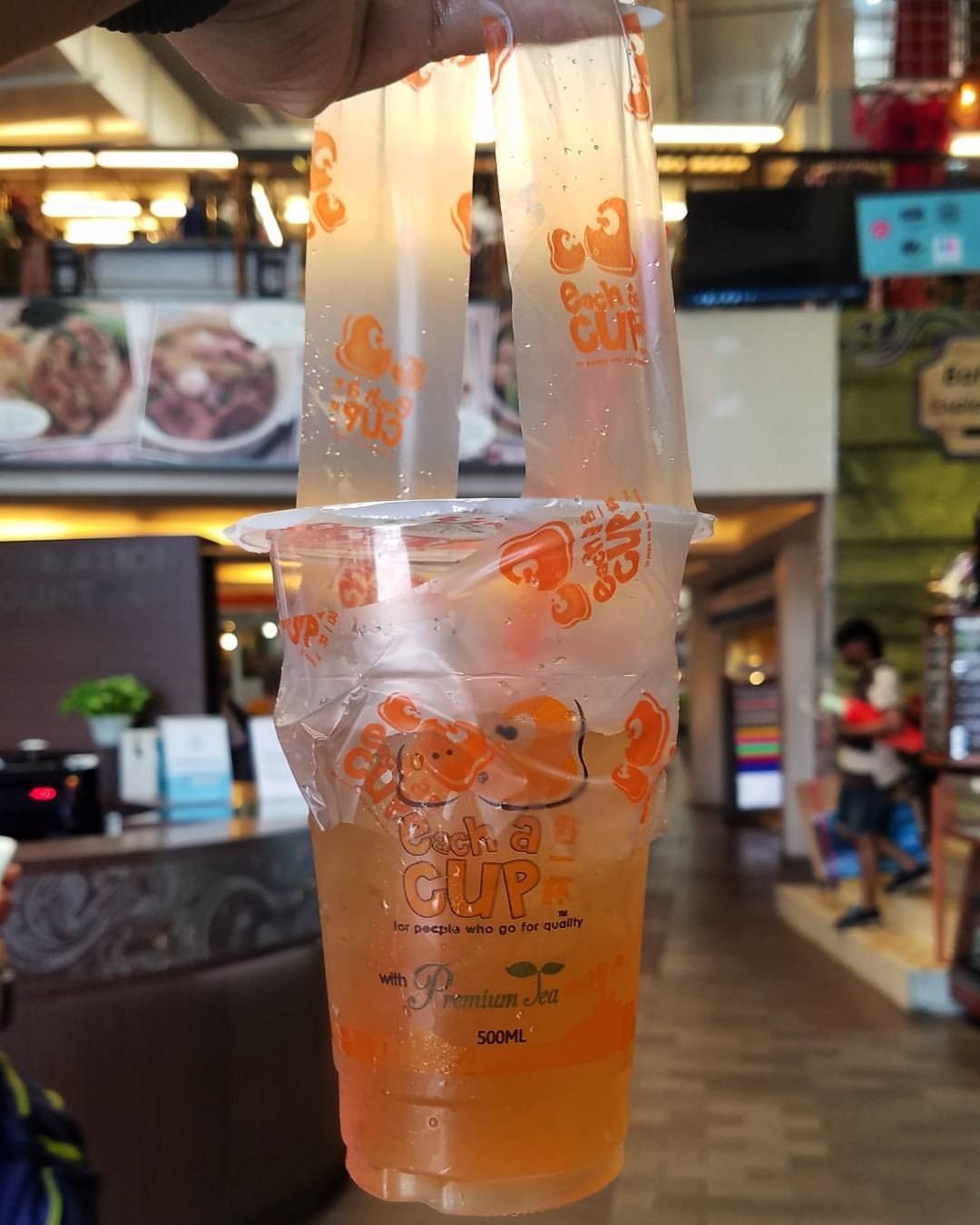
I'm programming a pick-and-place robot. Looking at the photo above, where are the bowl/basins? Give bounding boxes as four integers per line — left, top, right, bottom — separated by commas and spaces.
136, 316, 302, 460
0, 397, 52, 443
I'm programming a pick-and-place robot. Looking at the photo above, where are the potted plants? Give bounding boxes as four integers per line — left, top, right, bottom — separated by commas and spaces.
58, 674, 148, 748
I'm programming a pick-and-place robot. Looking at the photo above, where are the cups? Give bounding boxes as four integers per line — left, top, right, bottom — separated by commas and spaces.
230, 499, 714, 1213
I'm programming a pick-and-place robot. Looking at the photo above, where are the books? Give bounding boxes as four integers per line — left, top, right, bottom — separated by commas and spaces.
156, 716, 232, 821
249, 717, 303, 800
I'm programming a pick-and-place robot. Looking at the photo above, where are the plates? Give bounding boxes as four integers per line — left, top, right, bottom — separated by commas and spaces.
0, 296, 131, 443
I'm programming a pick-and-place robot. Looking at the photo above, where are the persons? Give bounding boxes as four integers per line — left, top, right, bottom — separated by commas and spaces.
830, 618, 929, 927
0, 195, 233, 297
0, 0, 621, 118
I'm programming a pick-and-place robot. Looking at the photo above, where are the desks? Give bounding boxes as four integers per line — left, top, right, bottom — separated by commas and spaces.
913, 750, 980, 962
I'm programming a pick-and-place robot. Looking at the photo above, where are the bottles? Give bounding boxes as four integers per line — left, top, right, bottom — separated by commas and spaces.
118, 714, 158, 809
948, 724, 969, 759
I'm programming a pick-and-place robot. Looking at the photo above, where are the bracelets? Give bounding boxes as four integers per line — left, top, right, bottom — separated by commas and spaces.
97, 0, 230, 34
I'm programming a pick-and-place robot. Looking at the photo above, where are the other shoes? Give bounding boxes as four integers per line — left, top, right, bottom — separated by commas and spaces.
884, 864, 936, 895
834, 903, 882, 929
